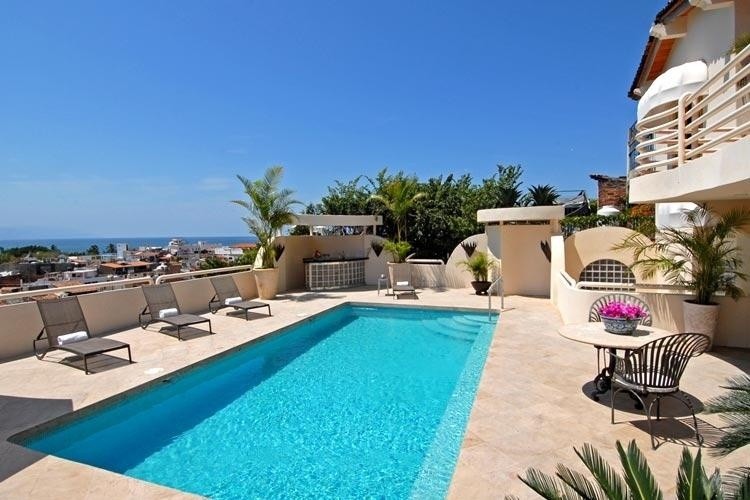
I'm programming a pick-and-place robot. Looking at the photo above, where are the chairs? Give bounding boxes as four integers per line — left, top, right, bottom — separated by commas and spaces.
554, 294, 710, 448
32, 274, 270, 376
387, 263, 417, 300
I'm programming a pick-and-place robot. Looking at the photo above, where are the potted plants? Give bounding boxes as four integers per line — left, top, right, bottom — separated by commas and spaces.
610, 202, 749, 353
455, 250, 499, 295
231, 164, 310, 300
